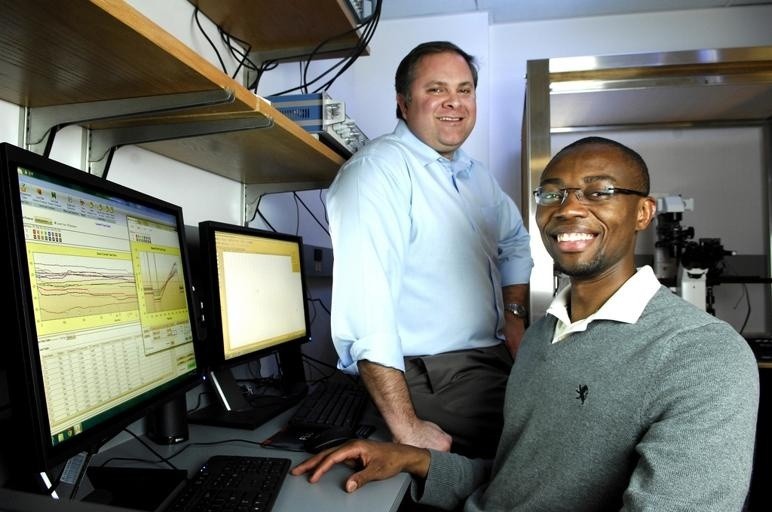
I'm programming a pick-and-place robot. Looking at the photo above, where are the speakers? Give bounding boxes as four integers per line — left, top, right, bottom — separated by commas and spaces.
145, 392, 190, 445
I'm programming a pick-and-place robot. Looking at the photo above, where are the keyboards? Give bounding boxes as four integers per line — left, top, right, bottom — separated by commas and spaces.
160, 455, 292, 512
287, 376, 367, 433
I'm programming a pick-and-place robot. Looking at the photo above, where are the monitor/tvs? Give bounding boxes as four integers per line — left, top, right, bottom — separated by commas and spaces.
0, 142, 209, 511
188, 220, 313, 431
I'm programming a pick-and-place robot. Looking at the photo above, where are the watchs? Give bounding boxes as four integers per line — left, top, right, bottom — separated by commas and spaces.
502, 300, 527, 319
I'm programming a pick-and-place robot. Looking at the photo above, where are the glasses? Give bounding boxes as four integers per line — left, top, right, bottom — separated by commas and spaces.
531, 184, 643, 207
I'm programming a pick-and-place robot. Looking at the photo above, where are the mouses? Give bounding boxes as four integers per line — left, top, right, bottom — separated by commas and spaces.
301, 428, 359, 454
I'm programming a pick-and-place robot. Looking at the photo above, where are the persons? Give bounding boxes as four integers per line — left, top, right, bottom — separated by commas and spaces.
287, 133, 762, 512
320, 39, 535, 462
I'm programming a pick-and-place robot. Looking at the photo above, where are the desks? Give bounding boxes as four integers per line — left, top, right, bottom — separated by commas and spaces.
0, 407, 412, 512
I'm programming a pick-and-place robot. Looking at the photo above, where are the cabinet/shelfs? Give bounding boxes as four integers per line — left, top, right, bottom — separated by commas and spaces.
0, 0, 370, 226
